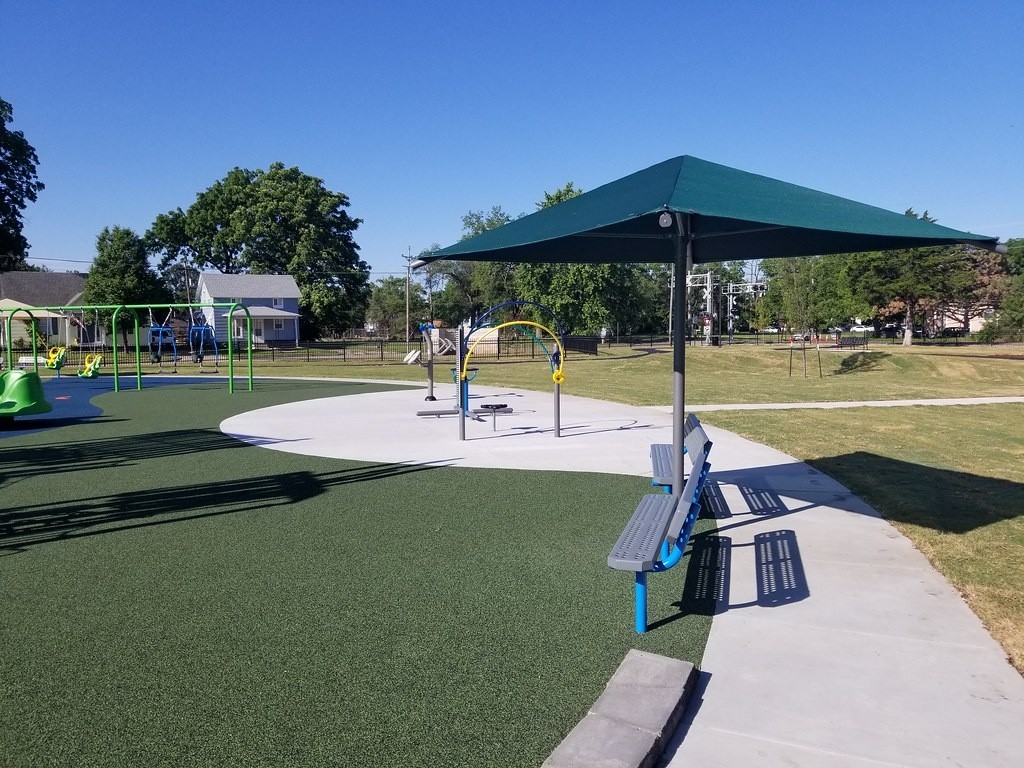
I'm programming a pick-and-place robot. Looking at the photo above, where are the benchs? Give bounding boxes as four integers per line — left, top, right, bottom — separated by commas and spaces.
650, 414, 712, 494
837, 335, 869, 349
607, 454, 712, 632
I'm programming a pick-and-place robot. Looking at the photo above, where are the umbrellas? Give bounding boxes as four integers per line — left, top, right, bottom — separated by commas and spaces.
0, 299, 67, 340
410, 154, 1008, 495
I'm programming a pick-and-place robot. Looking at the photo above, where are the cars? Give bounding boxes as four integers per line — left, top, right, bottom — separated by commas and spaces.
753, 323, 796, 335
850, 324, 876, 333
822, 321, 850, 335
792, 327, 821, 342
873, 322, 969, 339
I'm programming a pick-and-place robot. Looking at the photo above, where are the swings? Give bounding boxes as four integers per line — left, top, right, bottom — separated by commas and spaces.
147, 303, 178, 374
188, 303, 219, 374
77, 305, 105, 378
44, 305, 69, 370
